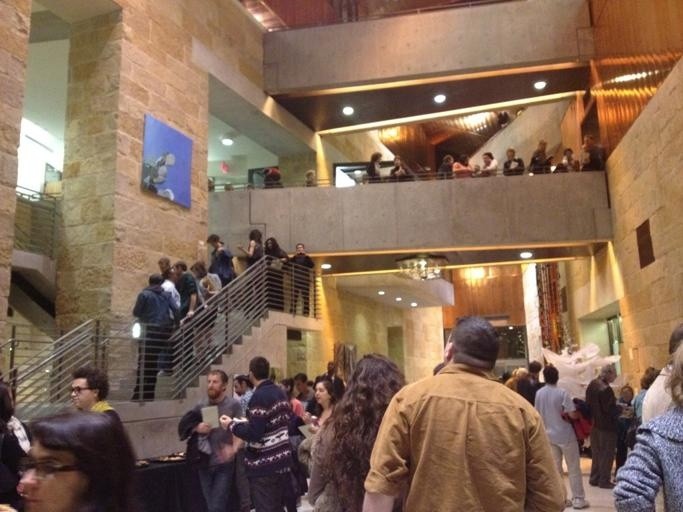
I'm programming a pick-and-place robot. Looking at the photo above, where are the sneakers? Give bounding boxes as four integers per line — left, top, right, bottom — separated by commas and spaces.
572, 497, 590, 508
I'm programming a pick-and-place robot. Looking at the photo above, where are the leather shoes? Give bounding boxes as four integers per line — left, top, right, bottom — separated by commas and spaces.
600, 482, 615, 489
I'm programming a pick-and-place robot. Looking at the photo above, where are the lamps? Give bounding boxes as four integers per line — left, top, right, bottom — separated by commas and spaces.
395, 254, 449, 281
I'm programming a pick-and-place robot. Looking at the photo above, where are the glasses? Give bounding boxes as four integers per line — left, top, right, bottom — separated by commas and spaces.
70, 387, 90, 392
19, 457, 78, 475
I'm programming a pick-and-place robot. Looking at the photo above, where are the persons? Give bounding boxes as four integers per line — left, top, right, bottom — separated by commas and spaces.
68, 368, 124, 426
274, 373, 305, 496
555, 147, 579, 172
533, 364, 588, 509
308, 378, 340, 434
530, 138, 553, 173
453, 153, 473, 170
583, 136, 606, 169
363, 316, 567, 511
293, 373, 311, 406
498, 367, 523, 389
184, 371, 245, 512
391, 156, 410, 177
367, 153, 383, 182
136, 275, 179, 403
286, 244, 314, 314
614, 339, 682, 512
158, 257, 177, 280
266, 237, 291, 264
262, 167, 282, 188
237, 230, 263, 268
173, 263, 196, 319
641, 323, 683, 421
614, 386, 634, 470
0, 382, 27, 511
517, 360, 544, 404
437, 155, 454, 176
158, 279, 181, 377
220, 359, 297, 512
205, 234, 236, 286
480, 152, 496, 175
635, 367, 659, 422
192, 261, 222, 366
585, 363, 625, 488
307, 351, 402, 511
19, 410, 141, 511
303, 170, 317, 187
314, 360, 345, 400
504, 147, 525, 175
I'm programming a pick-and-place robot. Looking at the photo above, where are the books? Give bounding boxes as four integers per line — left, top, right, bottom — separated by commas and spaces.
197, 405, 220, 432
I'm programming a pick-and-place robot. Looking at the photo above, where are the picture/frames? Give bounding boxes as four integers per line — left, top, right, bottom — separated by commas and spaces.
140, 116, 194, 210
